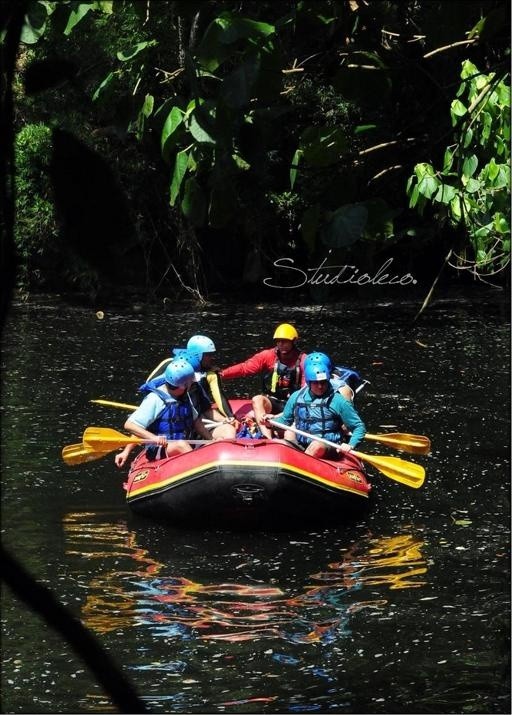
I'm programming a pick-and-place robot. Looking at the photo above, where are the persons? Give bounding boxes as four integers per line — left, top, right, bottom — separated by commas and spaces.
218, 323, 307, 439
259, 351, 367, 461
114, 334, 241, 469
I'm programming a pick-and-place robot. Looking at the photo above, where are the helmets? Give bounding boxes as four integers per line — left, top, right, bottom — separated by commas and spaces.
186, 335, 216, 362
165, 349, 200, 388
273, 323, 299, 343
303, 352, 332, 385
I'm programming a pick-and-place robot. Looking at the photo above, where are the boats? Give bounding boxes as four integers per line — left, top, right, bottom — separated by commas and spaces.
124, 400, 370, 519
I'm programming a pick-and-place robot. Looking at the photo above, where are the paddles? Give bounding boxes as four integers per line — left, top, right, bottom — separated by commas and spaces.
89, 399, 216, 424
82, 426, 213, 453
348, 432, 431, 455
61, 422, 223, 465
265, 417, 426, 489
